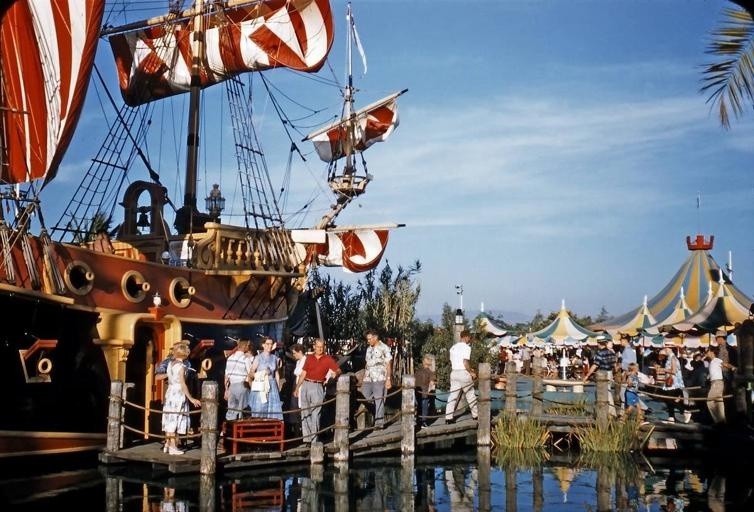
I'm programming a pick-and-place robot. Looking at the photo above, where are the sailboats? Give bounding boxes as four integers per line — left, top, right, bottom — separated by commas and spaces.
0, 0, 418, 491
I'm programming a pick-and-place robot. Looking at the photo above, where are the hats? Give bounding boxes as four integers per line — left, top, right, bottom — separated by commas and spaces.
663, 342, 677, 348
597, 336, 607, 343
715, 330, 729, 337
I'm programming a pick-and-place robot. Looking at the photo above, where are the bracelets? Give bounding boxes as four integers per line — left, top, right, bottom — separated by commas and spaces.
386, 376, 390, 380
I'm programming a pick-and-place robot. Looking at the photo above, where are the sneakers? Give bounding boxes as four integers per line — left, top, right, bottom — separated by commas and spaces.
164, 444, 185, 455
684, 411, 692, 424
640, 419, 650, 425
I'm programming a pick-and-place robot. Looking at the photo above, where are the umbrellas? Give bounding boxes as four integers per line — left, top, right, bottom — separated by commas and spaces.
551, 466, 582, 504
465, 266, 753, 350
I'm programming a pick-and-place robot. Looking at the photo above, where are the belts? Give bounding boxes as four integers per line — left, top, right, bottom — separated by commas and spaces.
304, 379, 322, 384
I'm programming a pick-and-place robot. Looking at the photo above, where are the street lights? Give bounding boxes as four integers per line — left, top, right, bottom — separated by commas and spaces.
455, 281, 463, 311
452, 309, 464, 343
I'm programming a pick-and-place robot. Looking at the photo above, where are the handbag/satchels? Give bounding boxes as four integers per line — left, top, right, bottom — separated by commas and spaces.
251, 380, 265, 391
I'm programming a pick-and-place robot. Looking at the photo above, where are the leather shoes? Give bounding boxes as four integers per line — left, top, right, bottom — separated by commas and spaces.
446, 420, 456, 425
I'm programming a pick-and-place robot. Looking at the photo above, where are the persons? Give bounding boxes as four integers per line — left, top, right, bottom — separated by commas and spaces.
356, 327, 395, 430
443, 329, 478, 425
580, 337, 611, 419
705, 346, 739, 424
413, 357, 437, 428
617, 335, 653, 418
288, 336, 342, 446
153, 338, 191, 453
158, 487, 188, 511
712, 318, 754, 425
444, 466, 477, 511
604, 339, 618, 417
210, 333, 307, 451
161, 343, 201, 455
618, 362, 644, 424
492, 344, 713, 383
659, 347, 692, 424
